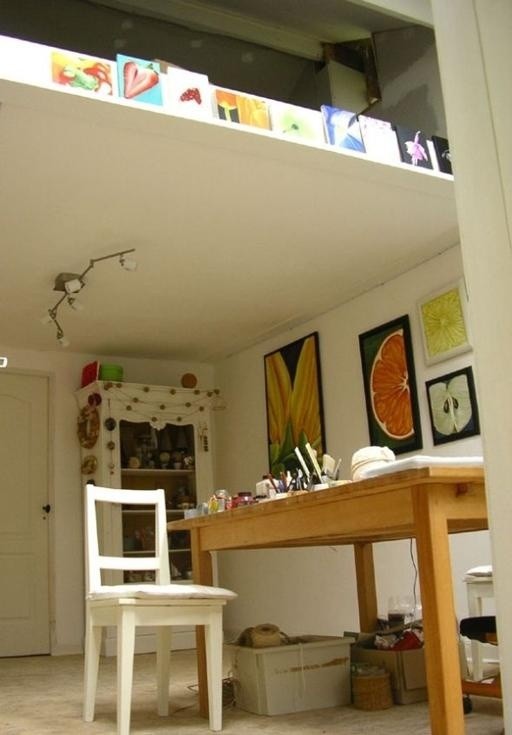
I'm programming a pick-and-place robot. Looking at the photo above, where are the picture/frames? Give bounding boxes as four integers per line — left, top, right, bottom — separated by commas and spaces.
356, 314, 424, 455
414, 278, 473, 369
262, 330, 327, 489
424, 365, 481, 447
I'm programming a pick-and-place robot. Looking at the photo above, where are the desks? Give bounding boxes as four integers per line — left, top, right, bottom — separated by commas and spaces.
161, 460, 505, 734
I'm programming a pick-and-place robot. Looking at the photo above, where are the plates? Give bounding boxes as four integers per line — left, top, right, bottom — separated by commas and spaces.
366, 454, 483, 478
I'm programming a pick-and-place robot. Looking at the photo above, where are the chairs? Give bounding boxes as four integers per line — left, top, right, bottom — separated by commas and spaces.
82, 483, 239, 734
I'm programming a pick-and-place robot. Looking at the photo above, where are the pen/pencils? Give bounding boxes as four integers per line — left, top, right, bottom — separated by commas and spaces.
267, 468, 304, 493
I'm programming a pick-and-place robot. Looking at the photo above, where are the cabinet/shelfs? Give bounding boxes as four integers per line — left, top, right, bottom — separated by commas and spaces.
71, 380, 232, 661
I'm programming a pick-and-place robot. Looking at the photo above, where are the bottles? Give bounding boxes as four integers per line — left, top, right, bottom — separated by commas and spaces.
208, 489, 232, 512
129, 437, 184, 470
235, 492, 255, 507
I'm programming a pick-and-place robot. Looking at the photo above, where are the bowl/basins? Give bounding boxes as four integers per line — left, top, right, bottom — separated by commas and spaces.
350, 446, 390, 481
100, 364, 124, 380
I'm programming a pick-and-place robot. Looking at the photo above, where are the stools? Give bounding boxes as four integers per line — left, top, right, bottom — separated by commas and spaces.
453, 610, 503, 717
458, 566, 497, 683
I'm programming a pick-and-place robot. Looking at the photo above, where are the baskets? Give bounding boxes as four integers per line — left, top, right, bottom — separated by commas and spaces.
350, 672, 395, 711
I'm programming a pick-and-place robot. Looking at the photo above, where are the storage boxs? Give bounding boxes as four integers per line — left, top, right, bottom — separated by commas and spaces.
348, 639, 432, 706
220, 625, 360, 720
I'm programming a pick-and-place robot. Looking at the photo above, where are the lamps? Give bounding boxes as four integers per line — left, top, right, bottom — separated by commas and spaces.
41, 247, 141, 351
